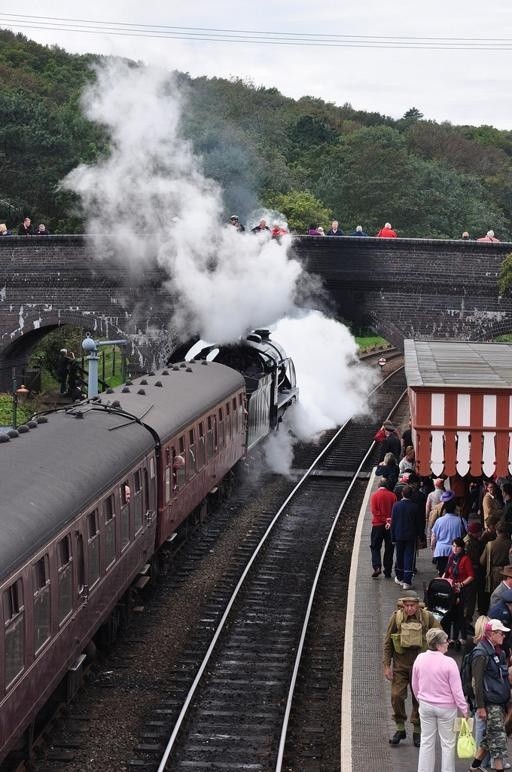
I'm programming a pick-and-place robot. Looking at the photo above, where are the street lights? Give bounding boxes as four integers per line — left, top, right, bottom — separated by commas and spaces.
10, 366, 28, 431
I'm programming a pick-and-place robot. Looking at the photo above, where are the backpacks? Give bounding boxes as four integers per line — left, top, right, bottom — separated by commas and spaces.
460, 640, 501, 699
395, 596, 430, 632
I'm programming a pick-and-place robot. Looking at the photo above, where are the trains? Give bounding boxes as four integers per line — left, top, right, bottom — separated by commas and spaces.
0, 330, 300, 766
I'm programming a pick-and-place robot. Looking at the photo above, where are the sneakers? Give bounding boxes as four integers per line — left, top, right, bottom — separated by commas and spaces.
402, 582, 415, 590
467, 764, 489, 772
490, 759, 511, 769
446, 624, 474, 654
394, 576, 404, 586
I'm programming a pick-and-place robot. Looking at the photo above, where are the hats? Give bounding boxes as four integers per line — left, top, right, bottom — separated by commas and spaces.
434, 478, 443, 488
398, 472, 412, 485
486, 618, 512, 633
406, 473, 424, 482
440, 490, 455, 503
499, 564, 512, 578
398, 590, 421, 602
384, 424, 395, 432
467, 521, 483, 533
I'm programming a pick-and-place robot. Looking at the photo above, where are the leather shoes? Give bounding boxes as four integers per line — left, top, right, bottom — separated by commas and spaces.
387, 728, 407, 745
385, 572, 391, 578
371, 568, 381, 577
412, 732, 420, 747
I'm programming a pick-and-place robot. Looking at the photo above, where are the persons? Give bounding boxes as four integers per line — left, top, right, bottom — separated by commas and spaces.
376, 221, 398, 237
251, 217, 271, 234
68, 351, 82, 392
327, 220, 346, 236
383, 589, 444, 746
349, 224, 368, 236
308, 227, 320, 235
16, 217, 35, 235
411, 627, 469, 772
0, 223, 14, 236
34, 223, 48, 235
317, 226, 325, 235
374, 418, 512, 650
459, 231, 471, 240
57, 348, 82, 394
228, 214, 245, 233
477, 229, 501, 242
271, 220, 289, 236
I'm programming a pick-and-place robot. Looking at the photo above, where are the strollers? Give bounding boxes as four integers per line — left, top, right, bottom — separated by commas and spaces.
421, 576, 458, 648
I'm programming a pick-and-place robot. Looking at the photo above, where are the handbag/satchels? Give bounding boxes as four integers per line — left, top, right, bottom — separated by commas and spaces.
499, 664, 509, 678
399, 621, 423, 650
373, 429, 385, 443
455, 717, 477, 760
